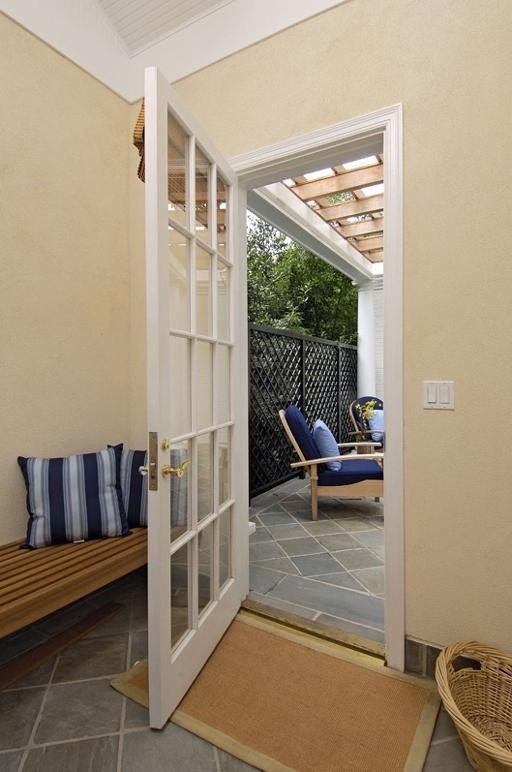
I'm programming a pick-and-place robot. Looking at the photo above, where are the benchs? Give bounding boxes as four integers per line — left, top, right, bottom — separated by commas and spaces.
1, 518, 202, 692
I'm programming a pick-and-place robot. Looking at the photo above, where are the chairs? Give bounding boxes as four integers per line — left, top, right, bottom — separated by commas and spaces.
347, 397, 384, 452
278, 405, 384, 520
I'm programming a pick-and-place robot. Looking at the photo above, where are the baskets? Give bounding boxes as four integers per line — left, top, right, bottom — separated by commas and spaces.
434, 639, 512, 771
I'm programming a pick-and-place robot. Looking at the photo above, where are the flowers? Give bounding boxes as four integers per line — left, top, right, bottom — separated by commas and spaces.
354, 399, 377, 440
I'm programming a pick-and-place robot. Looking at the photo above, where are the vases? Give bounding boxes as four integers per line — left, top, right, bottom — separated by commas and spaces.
356, 446, 371, 454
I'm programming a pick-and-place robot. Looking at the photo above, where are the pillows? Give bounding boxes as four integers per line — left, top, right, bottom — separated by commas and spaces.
16, 448, 132, 548
310, 419, 342, 472
107, 445, 187, 525
367, 409, 384, 442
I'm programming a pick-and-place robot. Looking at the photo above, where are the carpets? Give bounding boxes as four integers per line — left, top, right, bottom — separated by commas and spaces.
112, 607, 443, 771
